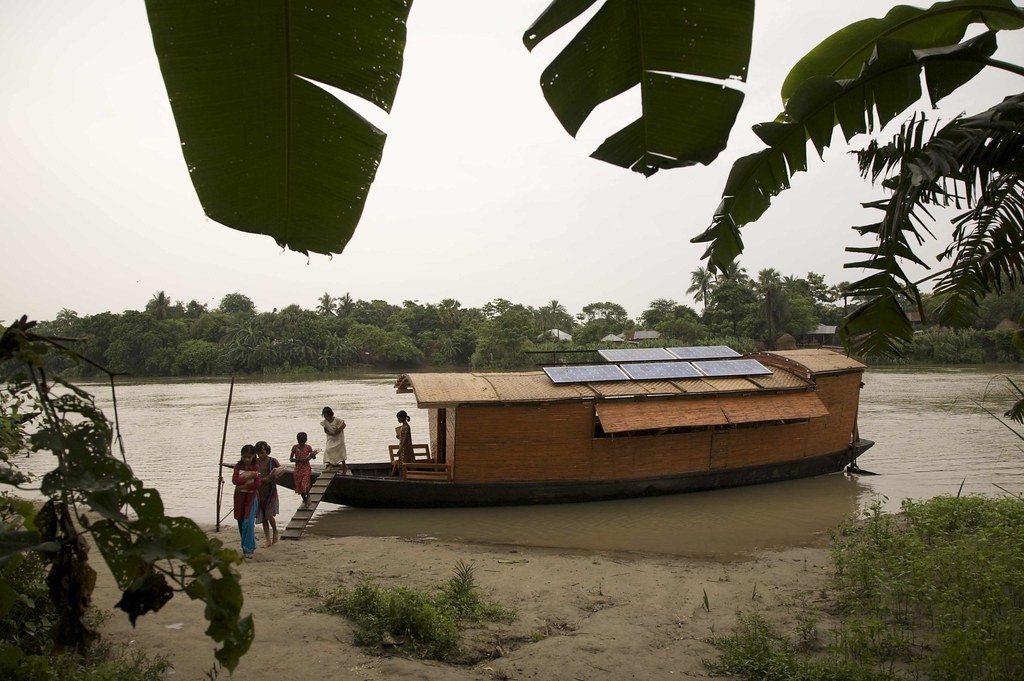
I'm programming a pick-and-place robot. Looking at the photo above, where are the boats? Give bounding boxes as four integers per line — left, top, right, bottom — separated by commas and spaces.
267, 345, 876, 511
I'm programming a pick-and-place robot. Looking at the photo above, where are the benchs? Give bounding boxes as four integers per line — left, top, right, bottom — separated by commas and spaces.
402, 463, 452, 482
389, 444, 435, 477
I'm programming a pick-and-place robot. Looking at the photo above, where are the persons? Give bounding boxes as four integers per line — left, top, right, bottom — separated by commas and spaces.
254, 441, 281, 549
232, 444, 261, 559
290, 432, 316, 508
396, 410, 415, 468
321, 407, 348, 474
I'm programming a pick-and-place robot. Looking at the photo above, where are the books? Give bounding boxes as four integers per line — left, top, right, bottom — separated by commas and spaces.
395, 426, 401, 441
239, 470, 253, 493
304, 448, 322, 462
269, 466, 286, 477
